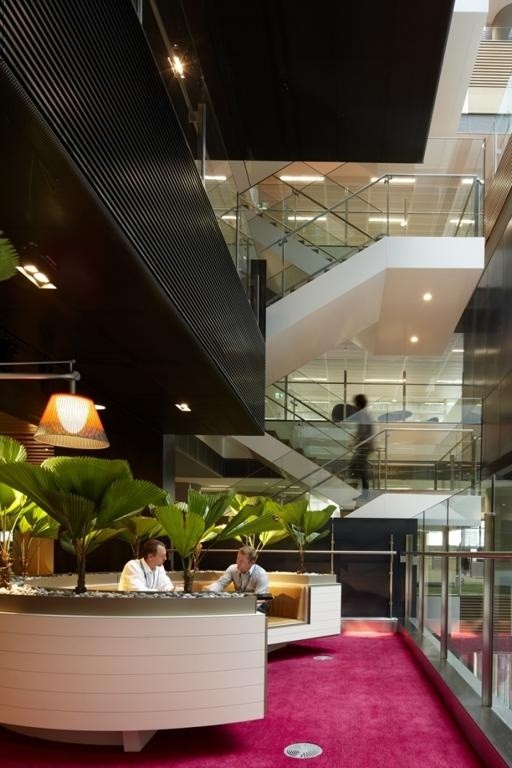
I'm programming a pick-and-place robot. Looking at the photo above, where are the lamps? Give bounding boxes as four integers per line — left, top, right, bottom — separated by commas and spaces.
0, 352, 110, 451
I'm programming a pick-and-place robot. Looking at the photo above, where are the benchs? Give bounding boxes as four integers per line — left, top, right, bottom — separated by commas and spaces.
0, 563, 343, 755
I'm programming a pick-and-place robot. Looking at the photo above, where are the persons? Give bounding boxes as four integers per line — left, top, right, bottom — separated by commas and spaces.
203, 546, 271, 616
348, 393, 377, 494
118, 538, 175, 592
